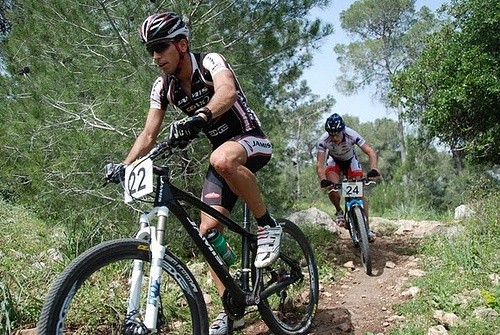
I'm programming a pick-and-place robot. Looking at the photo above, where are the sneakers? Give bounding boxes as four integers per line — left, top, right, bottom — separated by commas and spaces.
254, 216, 283, 268
209, 309, 244, 335
368, 230, 376, 243
335, 209, 345, 227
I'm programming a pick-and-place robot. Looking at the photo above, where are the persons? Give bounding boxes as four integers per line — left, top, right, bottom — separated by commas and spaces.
104, 11, 283, 335
316, 112, 380, 241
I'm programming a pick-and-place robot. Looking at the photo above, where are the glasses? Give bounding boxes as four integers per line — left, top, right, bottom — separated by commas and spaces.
146, 43, 170, 57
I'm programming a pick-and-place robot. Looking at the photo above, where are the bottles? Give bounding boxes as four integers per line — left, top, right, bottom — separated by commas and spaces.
205, 228, 237, 265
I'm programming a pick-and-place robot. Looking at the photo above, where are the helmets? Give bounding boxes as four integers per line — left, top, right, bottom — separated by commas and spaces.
140, 11, 189, 47
325, 113, 345, 136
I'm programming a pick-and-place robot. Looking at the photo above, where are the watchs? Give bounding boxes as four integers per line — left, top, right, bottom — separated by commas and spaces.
195, 106, 212, 122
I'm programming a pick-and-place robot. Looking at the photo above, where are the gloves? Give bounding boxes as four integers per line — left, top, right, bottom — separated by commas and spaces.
166, 115, 209, 150
367, 169, 381, 181
104, 162, 129, 184
320, 179, 333, 191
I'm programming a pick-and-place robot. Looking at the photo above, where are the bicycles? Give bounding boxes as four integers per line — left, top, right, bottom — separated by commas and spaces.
35, 132, 320, 335
317, 174, 383, 276
